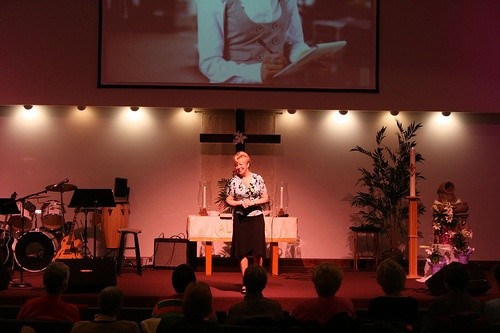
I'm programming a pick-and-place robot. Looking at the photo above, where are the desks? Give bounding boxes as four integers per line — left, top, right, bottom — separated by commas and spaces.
187, 214, 297, 276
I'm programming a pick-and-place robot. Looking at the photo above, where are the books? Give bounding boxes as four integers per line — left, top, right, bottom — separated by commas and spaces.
271, 41, 347, 80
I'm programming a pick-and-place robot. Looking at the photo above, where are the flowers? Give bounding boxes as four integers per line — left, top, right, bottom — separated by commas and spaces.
423, 201, 474, 264
232, 132, 247, 146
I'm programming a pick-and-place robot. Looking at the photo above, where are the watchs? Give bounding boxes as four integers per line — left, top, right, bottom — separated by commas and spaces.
251, 200, 256, 206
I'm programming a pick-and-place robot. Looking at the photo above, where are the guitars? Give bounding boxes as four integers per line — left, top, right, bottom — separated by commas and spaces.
0, 191, 18, 267
55, 209, 84, 259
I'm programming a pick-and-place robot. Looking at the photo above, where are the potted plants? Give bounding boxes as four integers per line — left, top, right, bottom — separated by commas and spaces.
344, 118, 427, 271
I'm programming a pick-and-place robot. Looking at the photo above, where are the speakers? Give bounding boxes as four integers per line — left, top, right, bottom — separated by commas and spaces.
154, 237, 197, 270
425, 262, 493, 297
55, 257, 118, 295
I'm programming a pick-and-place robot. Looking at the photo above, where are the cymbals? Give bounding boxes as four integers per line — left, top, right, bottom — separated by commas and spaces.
45, 182, 77, 192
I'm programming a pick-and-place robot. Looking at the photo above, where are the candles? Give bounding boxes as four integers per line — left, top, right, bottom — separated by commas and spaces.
203, 187, 206, 207
281, 186, 283, 209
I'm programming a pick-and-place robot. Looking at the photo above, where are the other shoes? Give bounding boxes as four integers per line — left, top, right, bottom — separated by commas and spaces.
242, 286, 246, 293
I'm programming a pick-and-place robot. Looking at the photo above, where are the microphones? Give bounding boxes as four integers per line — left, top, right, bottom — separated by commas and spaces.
51, 178, 69, 189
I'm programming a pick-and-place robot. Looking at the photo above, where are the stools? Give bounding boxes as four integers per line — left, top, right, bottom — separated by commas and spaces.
349, 225, 380, 271
116, 229, 143, 276
312, 20, 350, 60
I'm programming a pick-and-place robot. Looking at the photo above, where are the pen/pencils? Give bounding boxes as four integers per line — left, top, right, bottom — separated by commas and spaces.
257, 38, 285, 70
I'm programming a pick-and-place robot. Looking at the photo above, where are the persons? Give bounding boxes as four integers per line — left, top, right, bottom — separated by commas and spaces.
160, 282, 222, 333
224, 150, 268, 294
17, 262, 80, 322
196, 0, 334, 83
484, 263, 500, 324
368, 259, 419, 323
227, 265, 285, 320
70, 286, 140, 333
291, 262, 357, 323
425, 262, 485, 331
150, 263, 218, 321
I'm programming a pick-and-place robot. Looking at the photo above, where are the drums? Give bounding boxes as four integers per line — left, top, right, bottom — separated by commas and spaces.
13, 230, 57, 273
101, 199, 129, 250
41, 201, 65, 230
9, 200, 34, 229
